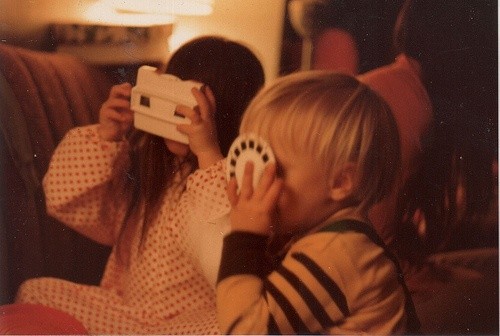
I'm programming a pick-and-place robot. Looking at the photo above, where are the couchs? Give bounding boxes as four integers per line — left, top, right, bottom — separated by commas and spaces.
0, 44, 112, 305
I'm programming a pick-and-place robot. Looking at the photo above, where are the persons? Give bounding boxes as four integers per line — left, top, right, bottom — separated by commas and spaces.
288, 0, 484, 302
15, 35, 266, 336
216, 70, 424, 336
